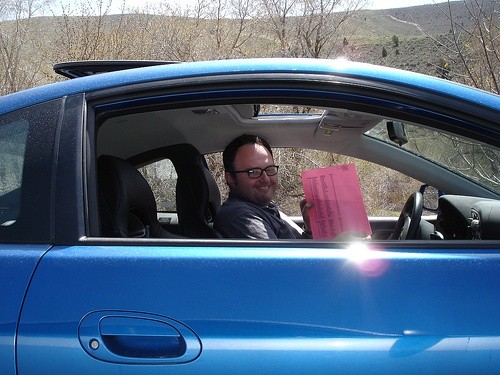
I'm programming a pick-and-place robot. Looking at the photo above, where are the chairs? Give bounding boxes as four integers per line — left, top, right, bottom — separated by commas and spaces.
96, 154, 178, 240
175, 164, 226, 239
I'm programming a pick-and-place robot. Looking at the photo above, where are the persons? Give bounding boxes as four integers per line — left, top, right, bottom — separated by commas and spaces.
213, 133, 312, 240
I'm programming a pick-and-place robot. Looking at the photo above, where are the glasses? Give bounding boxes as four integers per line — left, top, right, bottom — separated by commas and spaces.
229, 164, 279, 179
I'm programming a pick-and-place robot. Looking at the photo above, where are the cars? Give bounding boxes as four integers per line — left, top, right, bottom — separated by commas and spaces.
1, 60, 499, 374
